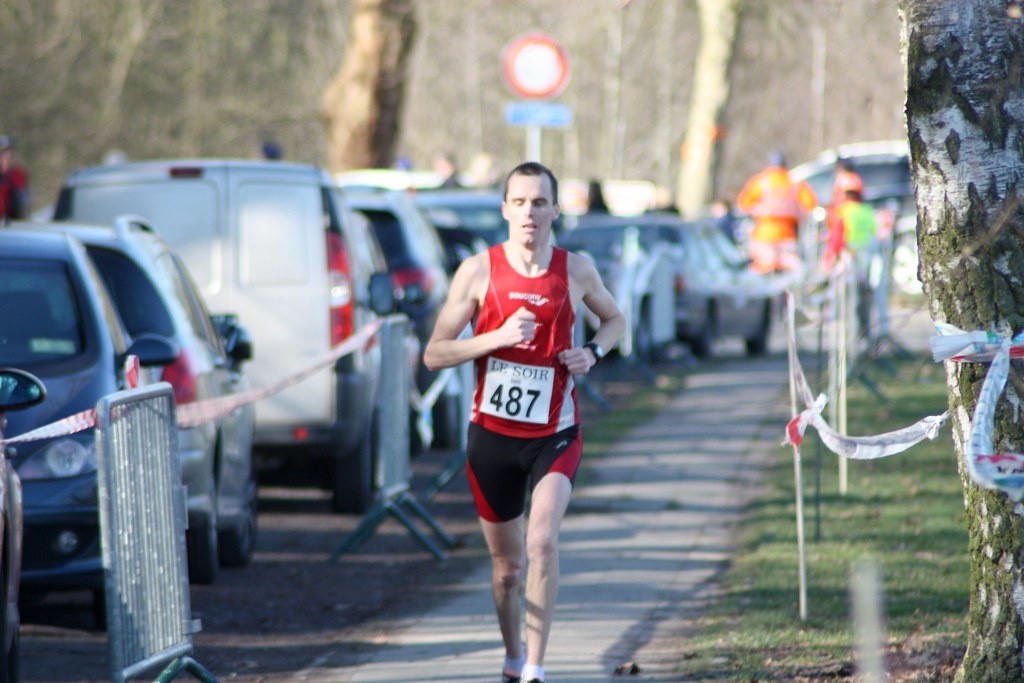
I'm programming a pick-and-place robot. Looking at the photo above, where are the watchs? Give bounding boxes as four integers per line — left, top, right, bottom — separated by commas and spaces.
583, 342, 604, 367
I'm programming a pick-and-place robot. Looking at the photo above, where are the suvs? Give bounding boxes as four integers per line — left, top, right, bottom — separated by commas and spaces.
1, 215, 262, 581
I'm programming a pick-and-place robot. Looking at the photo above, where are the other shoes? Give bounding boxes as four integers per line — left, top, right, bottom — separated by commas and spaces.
520, 676, 544, 683
503, 642, 526, 683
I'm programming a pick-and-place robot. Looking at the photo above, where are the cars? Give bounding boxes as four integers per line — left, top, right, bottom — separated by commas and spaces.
0, 364, 49, 683
1, 224, 182, 606
328, 144, 916, 444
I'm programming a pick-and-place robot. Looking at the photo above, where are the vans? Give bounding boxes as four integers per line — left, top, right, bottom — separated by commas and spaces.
59, 156, 406, 512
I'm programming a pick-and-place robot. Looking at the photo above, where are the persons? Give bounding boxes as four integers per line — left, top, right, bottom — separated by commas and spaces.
424, 162, 626, 683
740, 150, 817, 272
824, 157, 877, 341
712, 201, 738, 243
0, 134, 28, 221
